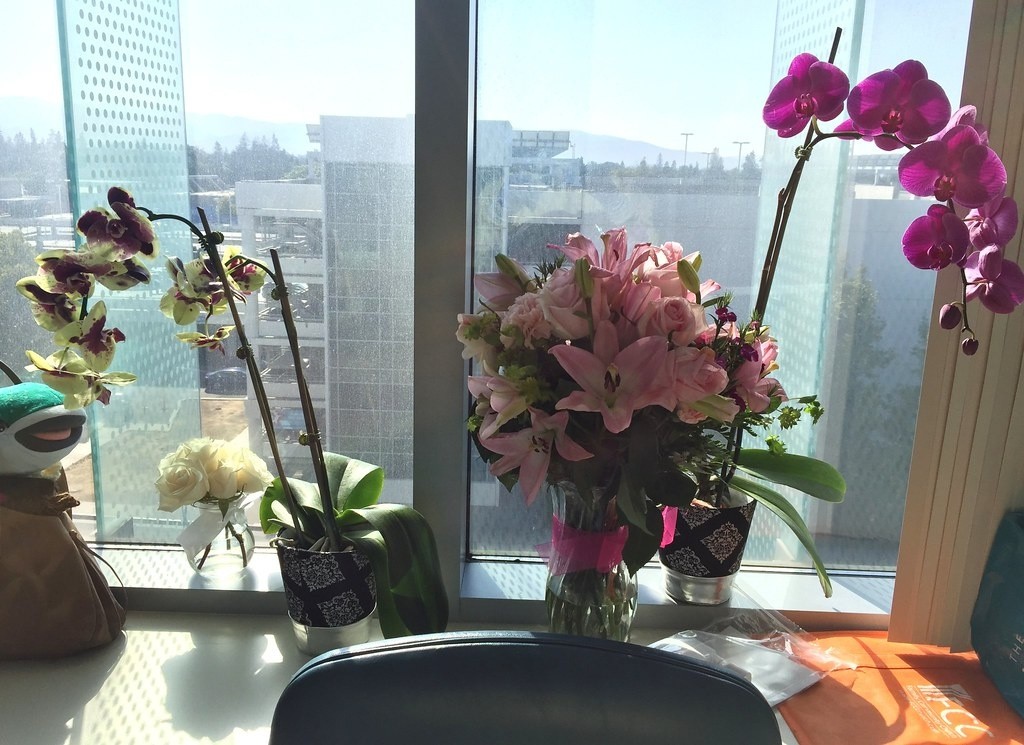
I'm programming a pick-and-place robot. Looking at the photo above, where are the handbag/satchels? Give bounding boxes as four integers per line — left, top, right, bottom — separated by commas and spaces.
0, 361, 127, 660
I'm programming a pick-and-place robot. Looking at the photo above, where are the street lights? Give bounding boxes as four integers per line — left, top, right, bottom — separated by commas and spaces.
680, 131, 694, 175
699, 150, 716, 184
732, 141, 753, 178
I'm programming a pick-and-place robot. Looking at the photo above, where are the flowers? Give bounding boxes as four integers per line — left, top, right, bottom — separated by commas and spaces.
14, 185, 448, 634
455, 223, 789, 640
699, 26, 1024, 598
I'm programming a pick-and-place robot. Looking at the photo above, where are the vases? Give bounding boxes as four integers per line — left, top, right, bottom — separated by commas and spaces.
545, 487, 638, 643
656, 472, 759, 606
276, 526, 378, 657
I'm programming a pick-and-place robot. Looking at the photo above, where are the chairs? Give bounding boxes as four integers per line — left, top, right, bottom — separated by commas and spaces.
269, 630, 781, 745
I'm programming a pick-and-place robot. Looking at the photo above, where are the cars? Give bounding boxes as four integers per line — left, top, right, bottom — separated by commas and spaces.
205, 367, 247, 392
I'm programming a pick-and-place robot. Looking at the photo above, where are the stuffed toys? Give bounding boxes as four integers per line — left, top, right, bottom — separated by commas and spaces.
0, 382, 128, 662
